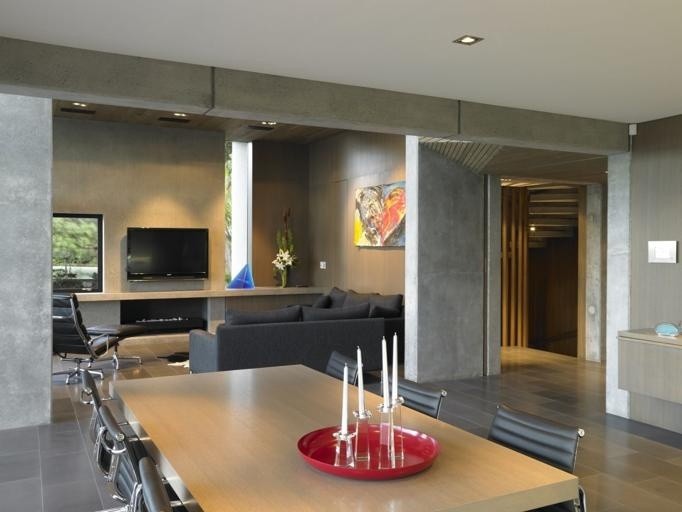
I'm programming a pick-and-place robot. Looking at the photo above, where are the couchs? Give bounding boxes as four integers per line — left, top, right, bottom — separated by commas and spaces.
185, 286, 408, 381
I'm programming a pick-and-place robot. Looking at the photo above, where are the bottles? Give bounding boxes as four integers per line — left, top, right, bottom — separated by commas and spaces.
351, 412, 374, 470
375, 397, 406, 471
332, 431, 357, 469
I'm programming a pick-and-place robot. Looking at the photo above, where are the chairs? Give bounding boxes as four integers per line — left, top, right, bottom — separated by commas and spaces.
51, 289, 160, 386
77, 370, 152, 458
323, 349, 364, 387
128, 454, 182, 512
374, 368, 450, 420
92, 407, 169, 512
486, 403, 590, 511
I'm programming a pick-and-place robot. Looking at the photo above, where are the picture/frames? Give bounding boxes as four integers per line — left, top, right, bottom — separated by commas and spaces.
52, 211, 104, 294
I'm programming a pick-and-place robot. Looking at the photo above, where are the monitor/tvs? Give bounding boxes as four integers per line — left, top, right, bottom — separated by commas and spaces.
127, 227, 208, 282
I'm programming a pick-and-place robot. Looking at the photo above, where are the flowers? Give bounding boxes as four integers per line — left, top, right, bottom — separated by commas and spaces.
269, 207, 310, 288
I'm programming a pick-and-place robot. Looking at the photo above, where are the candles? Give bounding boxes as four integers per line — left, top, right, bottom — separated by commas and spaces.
374, 333, 392, 410
333, 360, 353, 435
352, 343, 369, 418
389, 330, 402, 407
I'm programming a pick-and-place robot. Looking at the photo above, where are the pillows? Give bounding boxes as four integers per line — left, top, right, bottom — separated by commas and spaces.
223, 286, 404, 328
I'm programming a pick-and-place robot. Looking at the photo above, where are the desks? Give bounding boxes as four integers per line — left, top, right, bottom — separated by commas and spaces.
107, 360, 581, 511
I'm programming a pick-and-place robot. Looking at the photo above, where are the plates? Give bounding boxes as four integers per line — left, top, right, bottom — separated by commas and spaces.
296, 422, 441, 481
